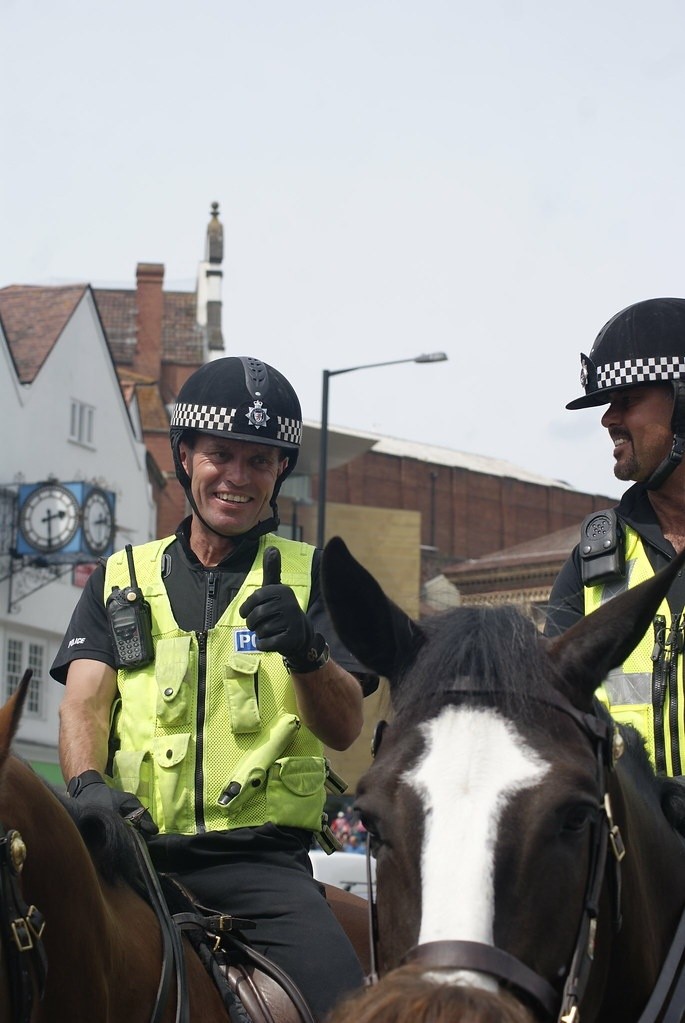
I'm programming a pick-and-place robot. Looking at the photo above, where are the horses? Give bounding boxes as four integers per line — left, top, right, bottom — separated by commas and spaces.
0, 535, 685, 1023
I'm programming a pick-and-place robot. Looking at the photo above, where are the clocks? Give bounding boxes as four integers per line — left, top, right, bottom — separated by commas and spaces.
17, 482, 115, 559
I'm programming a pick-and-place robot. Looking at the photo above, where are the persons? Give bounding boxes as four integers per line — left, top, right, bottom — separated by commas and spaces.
47, 354, 398, 1022
327, 802, 372, 856
547, 296, 684, 790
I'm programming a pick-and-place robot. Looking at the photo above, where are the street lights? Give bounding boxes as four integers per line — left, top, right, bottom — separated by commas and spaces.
313, 350, 451, 548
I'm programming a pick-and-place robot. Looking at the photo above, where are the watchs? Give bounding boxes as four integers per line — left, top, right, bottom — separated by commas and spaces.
281, 641, 332, 676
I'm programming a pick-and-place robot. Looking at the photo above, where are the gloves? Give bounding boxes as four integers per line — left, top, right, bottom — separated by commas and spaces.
238, 546, 325, 668
68, 769, 159, 886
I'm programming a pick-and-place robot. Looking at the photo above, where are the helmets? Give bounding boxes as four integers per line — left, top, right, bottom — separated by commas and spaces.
169, 356, 302, 449
565, 297, 685, 411
